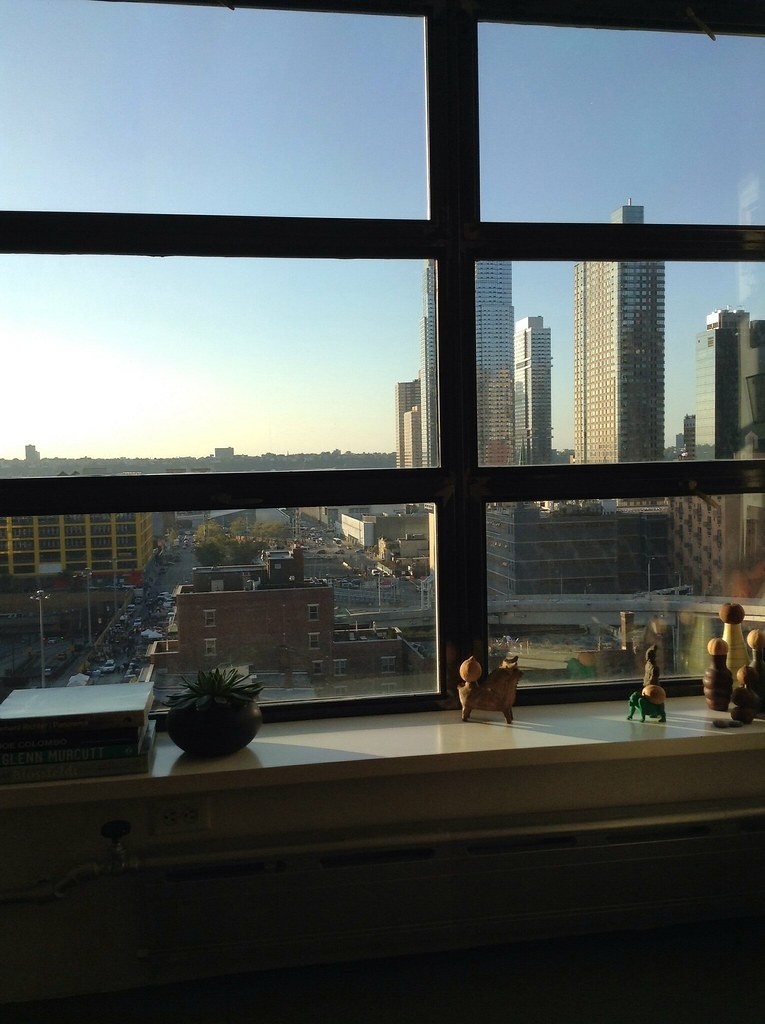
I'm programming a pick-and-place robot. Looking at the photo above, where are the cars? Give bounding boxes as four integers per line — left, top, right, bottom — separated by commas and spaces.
168, 529, 206, 555
280, 524, 426, 592
405, 631, 523, 668
64, 588, 177, 690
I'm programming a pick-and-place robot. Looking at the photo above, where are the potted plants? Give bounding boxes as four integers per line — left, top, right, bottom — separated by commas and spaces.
157, 669, 264, 758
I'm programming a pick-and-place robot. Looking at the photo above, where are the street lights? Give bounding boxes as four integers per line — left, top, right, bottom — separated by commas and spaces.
108, 557, 118, 614
645, 557, 656, 594
70, 567, 92, 655
30, 589, 54, 688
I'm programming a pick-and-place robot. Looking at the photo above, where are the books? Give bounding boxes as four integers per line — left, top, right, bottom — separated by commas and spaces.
1, 680, 157, 787
